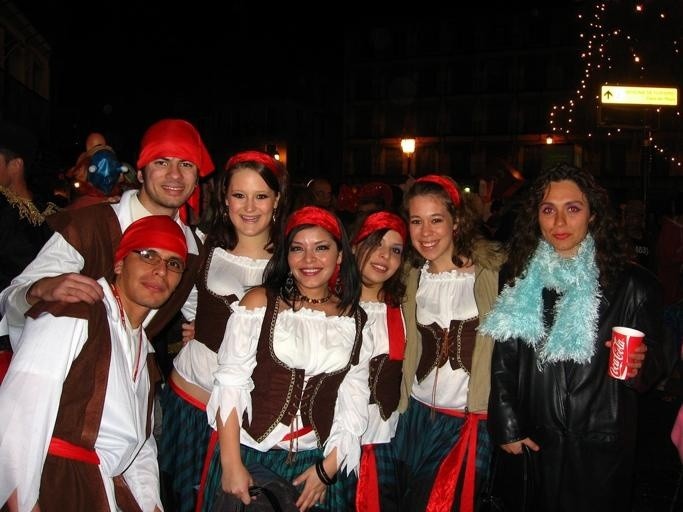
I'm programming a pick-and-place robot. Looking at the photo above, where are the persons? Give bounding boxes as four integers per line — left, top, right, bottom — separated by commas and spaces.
375, 164, 681, 510
0, 119, 408, 512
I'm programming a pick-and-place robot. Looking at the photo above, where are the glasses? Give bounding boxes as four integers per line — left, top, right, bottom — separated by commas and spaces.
133, 249, 185, 274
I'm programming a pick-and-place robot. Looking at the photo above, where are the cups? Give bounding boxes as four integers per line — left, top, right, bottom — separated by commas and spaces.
607, 325, 646, 381
